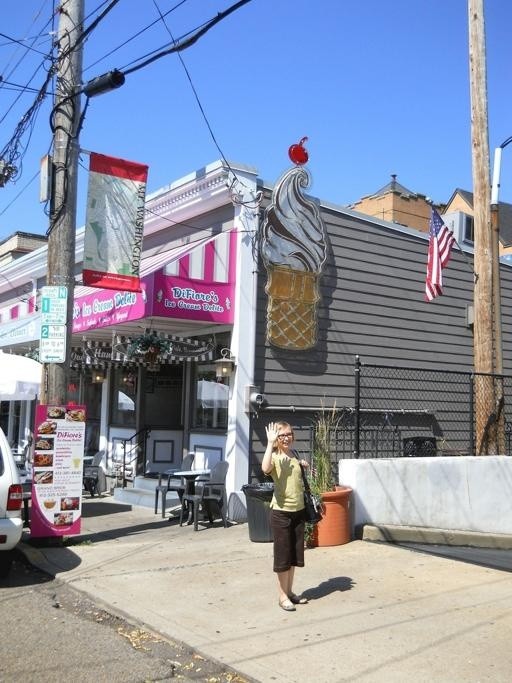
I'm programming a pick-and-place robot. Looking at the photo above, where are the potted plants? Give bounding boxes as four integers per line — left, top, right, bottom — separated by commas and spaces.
307, 398, 353, 547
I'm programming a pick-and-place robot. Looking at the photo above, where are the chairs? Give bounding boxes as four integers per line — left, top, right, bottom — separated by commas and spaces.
155, 454, 195, 518
12, 443, 105, 497
180, 460, 228, 531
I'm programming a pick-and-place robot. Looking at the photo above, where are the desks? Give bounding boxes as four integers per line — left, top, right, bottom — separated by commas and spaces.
174, 469, 211, 525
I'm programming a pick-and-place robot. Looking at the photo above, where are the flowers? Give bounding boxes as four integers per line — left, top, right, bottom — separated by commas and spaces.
128, 334, 171, 357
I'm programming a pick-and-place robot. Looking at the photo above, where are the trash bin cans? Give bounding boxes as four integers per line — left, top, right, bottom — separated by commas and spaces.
243, 483, 274, 542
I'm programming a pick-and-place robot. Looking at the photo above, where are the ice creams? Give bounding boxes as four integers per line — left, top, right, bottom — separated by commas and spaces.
259, 135, 327, 350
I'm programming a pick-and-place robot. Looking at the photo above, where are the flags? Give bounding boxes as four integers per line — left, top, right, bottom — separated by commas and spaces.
424, 205, 456, 304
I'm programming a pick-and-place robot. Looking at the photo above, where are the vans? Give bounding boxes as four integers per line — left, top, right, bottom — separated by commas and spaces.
0, 427, 23, 551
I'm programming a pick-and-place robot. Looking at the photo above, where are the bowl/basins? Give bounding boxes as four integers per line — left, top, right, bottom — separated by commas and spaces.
44, 501, 56, 508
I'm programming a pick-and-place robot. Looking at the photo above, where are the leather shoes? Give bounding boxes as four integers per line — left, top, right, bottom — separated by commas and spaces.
289, 593, 308, 604
279, 597, 296, 611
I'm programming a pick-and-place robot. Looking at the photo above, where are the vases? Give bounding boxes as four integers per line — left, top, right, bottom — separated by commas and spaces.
140, 351, 156, 362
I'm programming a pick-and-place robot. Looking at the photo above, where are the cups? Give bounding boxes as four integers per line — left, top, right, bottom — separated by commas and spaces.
73, 458, 81, 468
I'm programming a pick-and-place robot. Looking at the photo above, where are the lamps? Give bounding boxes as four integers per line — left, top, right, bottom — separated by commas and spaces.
92, 363, 107, 384
213, 347, 236, 377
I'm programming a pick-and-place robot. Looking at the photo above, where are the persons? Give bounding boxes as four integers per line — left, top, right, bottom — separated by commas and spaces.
261, 419, 309, 611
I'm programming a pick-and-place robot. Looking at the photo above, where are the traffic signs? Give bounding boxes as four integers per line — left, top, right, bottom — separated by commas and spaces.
40, 325, 67, 363
41, 286, 68, 326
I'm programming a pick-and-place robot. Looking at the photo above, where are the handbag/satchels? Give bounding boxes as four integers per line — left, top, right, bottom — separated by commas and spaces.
304, 490, 323, 524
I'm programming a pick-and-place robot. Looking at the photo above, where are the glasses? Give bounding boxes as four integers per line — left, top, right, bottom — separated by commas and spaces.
277, 432, 292, 440
